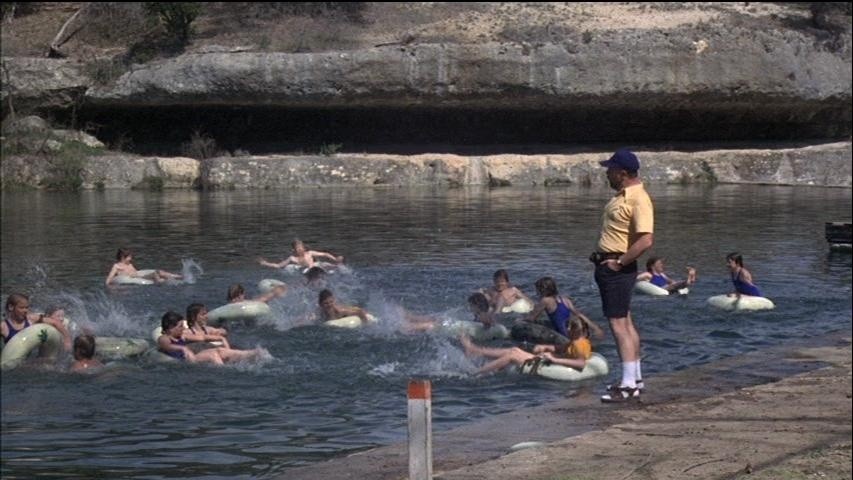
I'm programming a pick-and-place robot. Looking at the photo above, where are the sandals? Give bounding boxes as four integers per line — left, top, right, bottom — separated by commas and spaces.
601, 386, 640, 403
606, 379, 644, 393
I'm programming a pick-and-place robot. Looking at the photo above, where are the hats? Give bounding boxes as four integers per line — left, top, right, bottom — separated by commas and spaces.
599, 151, 639, 171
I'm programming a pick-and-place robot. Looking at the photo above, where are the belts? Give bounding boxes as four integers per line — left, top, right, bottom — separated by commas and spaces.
599, 252, 623, 259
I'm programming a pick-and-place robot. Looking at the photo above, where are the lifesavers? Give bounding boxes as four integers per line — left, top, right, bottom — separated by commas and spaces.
707, 293, 775, 311
491, 297, 533, 313
441, 317, 509, 344
144, 342, 218, 369
0, 322, 67, 374
285, 262, 336, 275
90, 336, 150, 359
32, 312, 82, 345
634, 281, 691, 297
511, 319, 570, 353
111, 267, 170, 286
151, 318, 226, 349
522, 349, 608, 381
205, 299, 271, 328
324, 313, 379, 331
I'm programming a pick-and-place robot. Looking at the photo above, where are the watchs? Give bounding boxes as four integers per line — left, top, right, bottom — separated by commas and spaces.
615, 256, 625, 267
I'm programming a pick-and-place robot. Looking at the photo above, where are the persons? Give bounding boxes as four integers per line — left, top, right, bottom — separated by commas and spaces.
588, 147, 654, 404
726, 252, 762, 295
635, 255, 697, 292
0, 282, 287, 375
455, 267, 605, 377
105, 246, 183, 285
256, 238, 344, 272
318, 288, 433, 333
